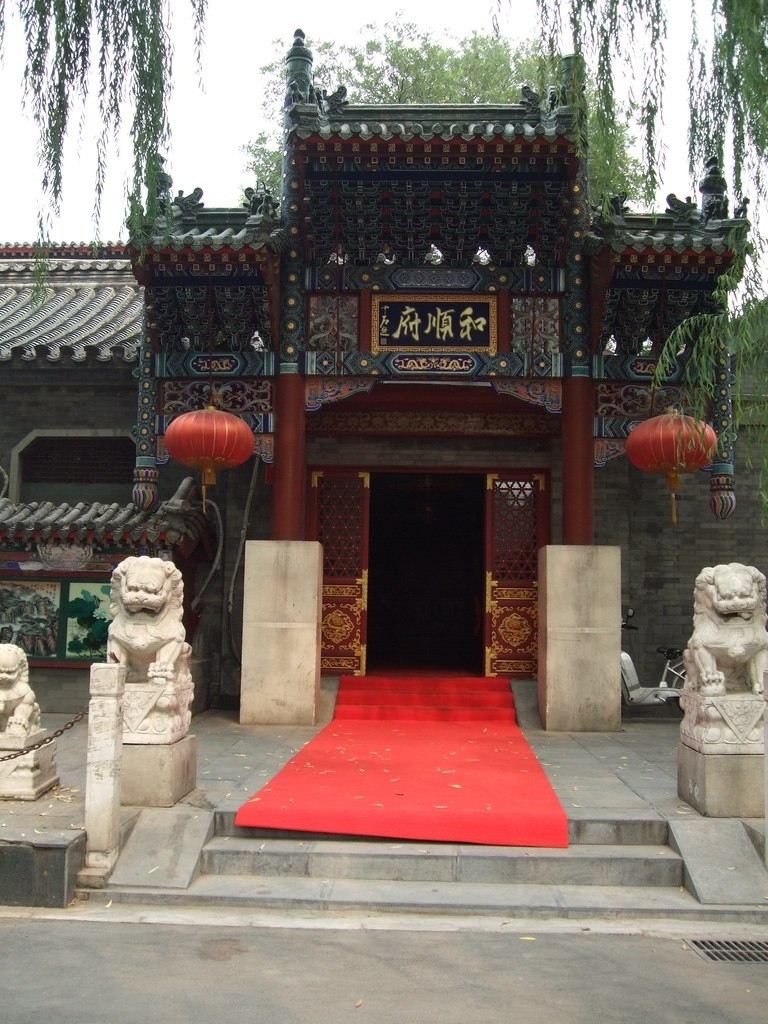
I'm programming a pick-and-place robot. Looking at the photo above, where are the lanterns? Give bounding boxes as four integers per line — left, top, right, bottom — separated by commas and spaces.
625, 409, 718, 523
165, 405, 255, 513
